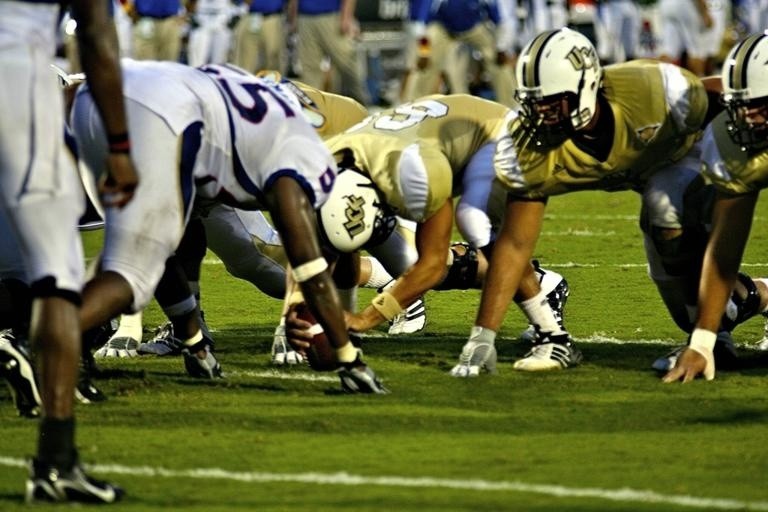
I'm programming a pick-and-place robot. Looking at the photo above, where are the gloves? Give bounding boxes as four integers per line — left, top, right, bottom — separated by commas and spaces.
266, 317, 308, 367
448, 325, 502, 379
92, 309, 147, 362
330, 341, 393, 397
176, 331, 231, 386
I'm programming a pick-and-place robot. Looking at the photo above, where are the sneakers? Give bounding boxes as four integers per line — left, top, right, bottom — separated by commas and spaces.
22, 453, 134, 510
519, 258, 570, 341
135, 314, 214, 357
511, 335, 586, 375
758, 327, 768, 351
73, 372, 109, 406
0, 326, 43, 420
648, 327, 741, 376
376, 275, 428, 337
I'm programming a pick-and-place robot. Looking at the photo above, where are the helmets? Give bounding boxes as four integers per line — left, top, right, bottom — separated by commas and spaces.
315, 167, 394, 255
509, 24, 606, 150
718, 32, 768, 154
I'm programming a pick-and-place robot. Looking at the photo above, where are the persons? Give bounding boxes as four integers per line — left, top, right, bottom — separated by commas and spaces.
0, 0, 768, 507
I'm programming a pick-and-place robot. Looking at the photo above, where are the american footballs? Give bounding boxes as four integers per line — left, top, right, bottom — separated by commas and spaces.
296, 301, 347, 372
